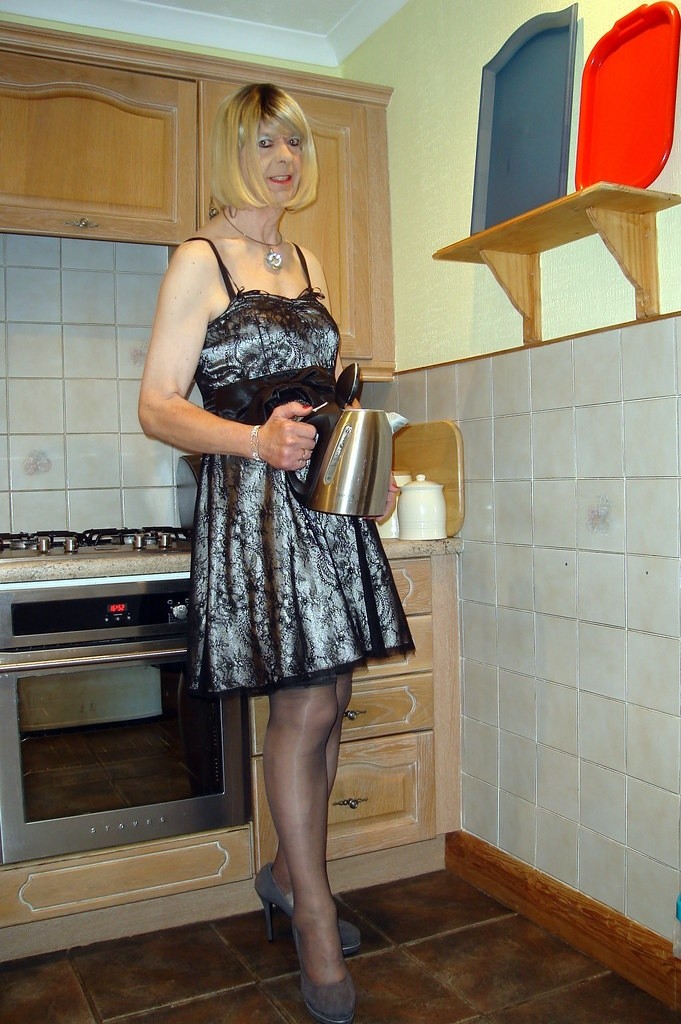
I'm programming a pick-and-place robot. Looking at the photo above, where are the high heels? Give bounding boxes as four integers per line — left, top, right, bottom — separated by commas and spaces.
254, 862, 361, 955
292, 920, 356, 1024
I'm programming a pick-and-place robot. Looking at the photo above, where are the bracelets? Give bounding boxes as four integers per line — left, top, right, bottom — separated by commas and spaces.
250, 425, 265, 463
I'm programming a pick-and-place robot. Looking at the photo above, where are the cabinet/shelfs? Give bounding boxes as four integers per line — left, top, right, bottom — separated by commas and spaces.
0, 537, 464, 962
0, 11, 394, 382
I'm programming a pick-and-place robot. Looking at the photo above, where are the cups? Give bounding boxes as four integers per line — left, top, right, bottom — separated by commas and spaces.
398, 475, 447, 541
376, 475, 412, 538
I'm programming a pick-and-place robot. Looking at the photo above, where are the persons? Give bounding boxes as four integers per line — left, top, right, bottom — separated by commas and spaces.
136, 80, 414, 1024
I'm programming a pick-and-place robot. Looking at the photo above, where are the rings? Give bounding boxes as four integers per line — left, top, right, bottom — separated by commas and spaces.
301, 449, 308, 460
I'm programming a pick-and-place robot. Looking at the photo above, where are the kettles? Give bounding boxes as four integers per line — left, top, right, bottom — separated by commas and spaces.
284, 362, 391, 515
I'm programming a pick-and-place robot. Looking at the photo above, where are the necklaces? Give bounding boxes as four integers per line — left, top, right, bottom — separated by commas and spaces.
223, 208, 284, 271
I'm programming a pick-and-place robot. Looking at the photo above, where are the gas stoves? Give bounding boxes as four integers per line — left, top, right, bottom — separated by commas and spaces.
0, 525, 196, 583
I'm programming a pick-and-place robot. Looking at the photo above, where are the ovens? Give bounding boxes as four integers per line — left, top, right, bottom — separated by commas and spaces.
0, 578, 245, 867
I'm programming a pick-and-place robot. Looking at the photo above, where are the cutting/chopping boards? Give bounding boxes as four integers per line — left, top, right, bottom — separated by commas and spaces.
392, 420, 465, 538
